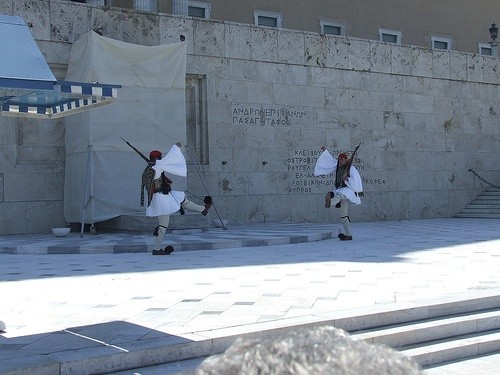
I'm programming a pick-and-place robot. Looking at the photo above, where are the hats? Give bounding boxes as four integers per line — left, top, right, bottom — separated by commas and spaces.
337, 154, 346, 159
150, 151, 162, 158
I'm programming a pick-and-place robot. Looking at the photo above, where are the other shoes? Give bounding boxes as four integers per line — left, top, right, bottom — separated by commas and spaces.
201, 196, 212, 216
338, 234, 352, 240
325, 192, 334, 207
153, 246, 173, 255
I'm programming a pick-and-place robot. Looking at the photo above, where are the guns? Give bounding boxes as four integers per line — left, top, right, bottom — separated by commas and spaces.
119, 135, 174, 184
343, 141, 362, 181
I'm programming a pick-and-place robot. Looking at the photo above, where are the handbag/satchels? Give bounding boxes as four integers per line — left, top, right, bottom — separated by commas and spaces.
161, 181, 168, 194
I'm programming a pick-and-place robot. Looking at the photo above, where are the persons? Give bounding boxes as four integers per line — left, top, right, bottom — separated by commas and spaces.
315, 142, 363, 240
141, 143, 212, 254
